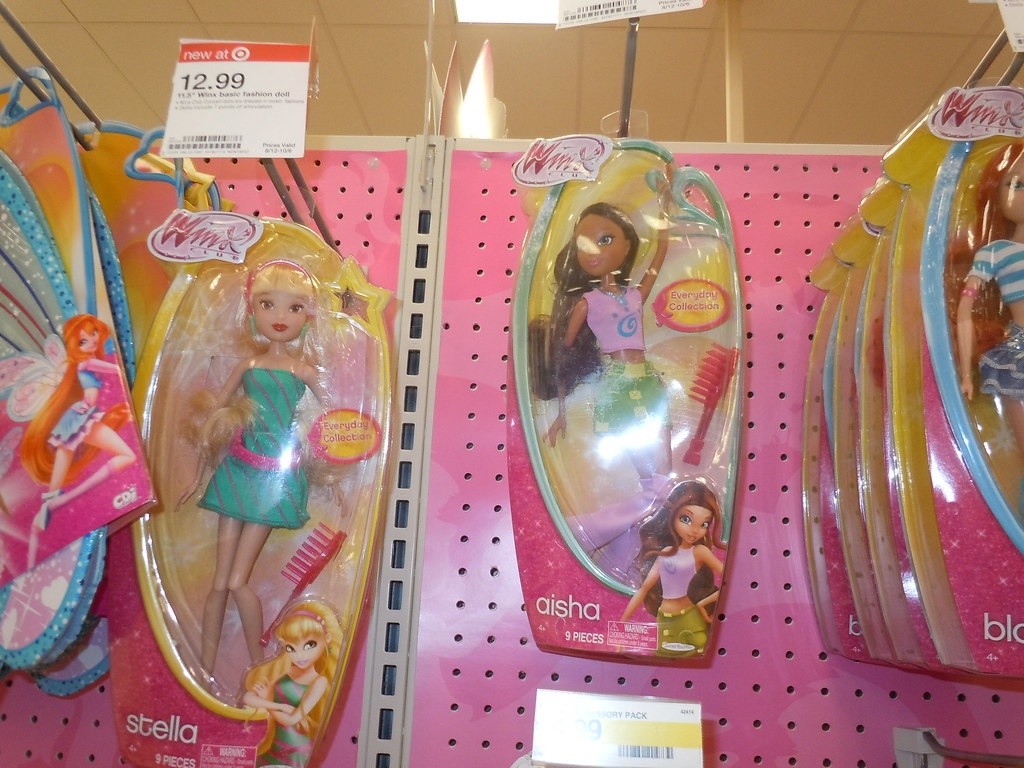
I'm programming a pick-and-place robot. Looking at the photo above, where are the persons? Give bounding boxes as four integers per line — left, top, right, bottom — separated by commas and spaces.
172, 256, 377, 704
949, 140, 1024, 468
527, 164, 703, 499
